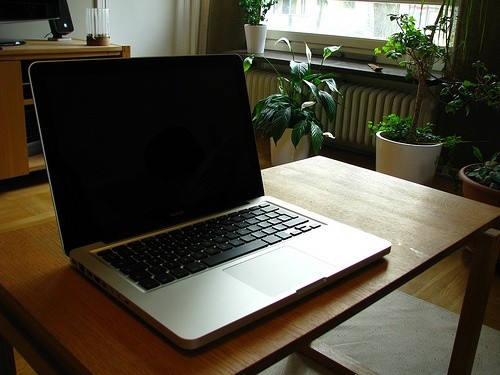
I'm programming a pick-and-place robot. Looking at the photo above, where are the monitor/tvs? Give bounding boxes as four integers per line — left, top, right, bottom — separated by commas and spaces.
0, 0, 60, 46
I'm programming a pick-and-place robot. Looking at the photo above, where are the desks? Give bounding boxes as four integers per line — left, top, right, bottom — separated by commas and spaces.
0, 154, 500, 375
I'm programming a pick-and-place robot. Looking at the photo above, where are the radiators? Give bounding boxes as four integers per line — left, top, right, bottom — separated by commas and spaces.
244, 69, 436, 154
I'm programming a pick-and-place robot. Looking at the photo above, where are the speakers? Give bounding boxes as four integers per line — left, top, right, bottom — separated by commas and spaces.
48, 0, 74, 40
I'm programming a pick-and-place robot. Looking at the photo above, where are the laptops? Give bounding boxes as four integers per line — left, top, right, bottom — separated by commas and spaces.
28, 53, 392, 350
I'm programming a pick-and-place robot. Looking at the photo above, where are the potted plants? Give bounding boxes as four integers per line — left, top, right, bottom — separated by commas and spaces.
235, 0, 279, 54
367, 14, 500, 208
243, 37, 345, 166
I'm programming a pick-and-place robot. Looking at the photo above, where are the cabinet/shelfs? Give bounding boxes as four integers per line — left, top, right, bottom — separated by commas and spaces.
0, 37, 131, 181
